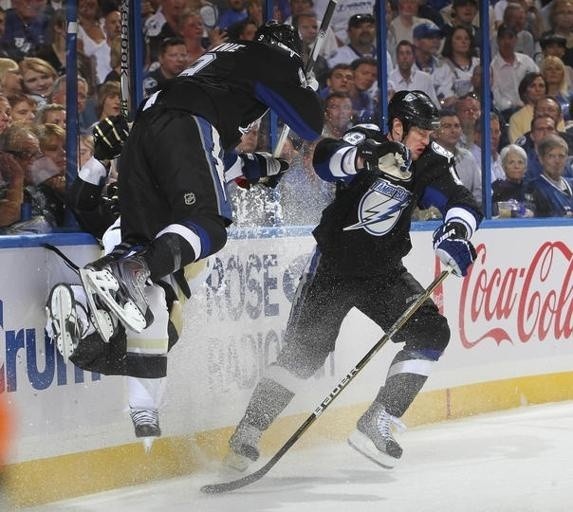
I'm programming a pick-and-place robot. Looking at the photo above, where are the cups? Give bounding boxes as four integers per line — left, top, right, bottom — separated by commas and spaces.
497, 201, 514, 217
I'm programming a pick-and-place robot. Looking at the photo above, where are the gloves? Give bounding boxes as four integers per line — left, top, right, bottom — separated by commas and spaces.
91, 113, 130, 160
432, 222, 477, 280
355, 137, 414, 183
233, 151, 290, 190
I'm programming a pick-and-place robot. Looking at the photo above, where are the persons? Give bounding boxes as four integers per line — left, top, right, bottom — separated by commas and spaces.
44, 19, 327, 379
224, 90, 485, 472
1, 0, 573, 242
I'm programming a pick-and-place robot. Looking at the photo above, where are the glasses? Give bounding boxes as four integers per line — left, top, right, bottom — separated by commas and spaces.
458, 92, 478, 101
530, 124, 555, 132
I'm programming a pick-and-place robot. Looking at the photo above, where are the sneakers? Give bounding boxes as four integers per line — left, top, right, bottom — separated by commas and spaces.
228, 420, 263, 463
47, 280, 81, 359
128, 407, 160, 438
357, 402, 403, 461
84, 244, 153, 329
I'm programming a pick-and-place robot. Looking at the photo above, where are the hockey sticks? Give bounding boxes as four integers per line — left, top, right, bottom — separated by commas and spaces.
199, 266, 453, 495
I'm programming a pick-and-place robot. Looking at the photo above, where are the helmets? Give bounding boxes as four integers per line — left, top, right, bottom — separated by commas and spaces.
252, 19, 307, 60
387, 90, 441, 138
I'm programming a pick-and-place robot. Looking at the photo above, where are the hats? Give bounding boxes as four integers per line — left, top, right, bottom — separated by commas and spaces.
348, 12, 377, 29
540, 32, 567, 49
497, 23, 517, 39
412, 23, 445, 40
